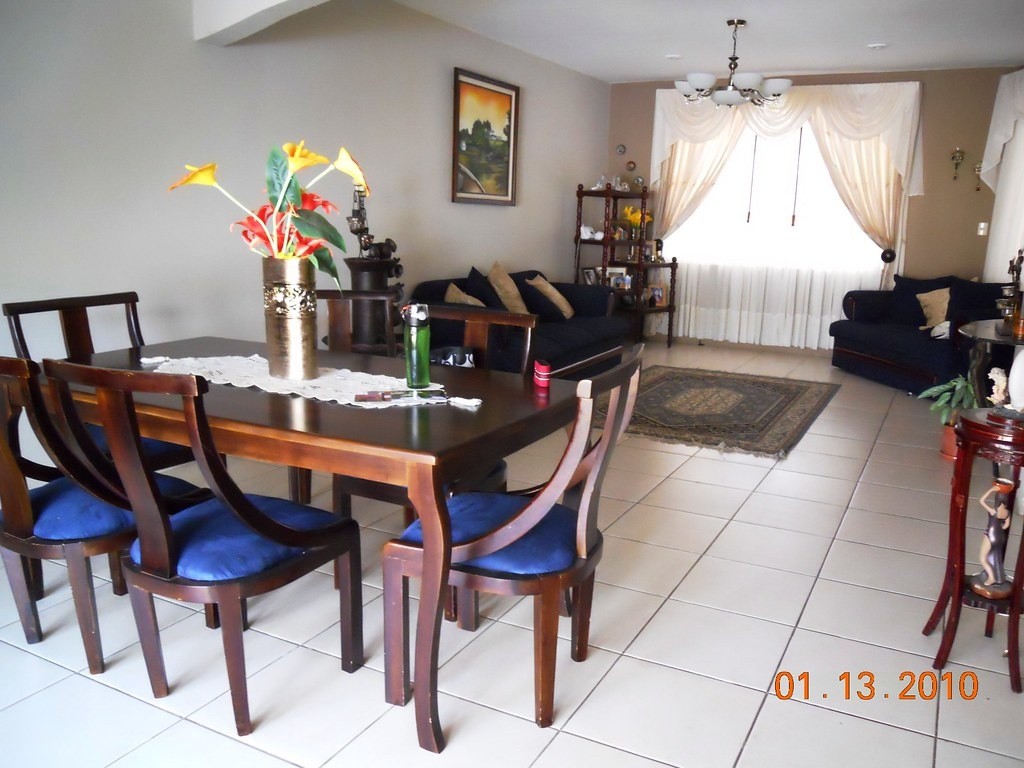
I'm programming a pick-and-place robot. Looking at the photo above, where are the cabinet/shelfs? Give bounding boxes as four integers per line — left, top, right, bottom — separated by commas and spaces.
613, 251, 678, 347
570, 182, 655, 343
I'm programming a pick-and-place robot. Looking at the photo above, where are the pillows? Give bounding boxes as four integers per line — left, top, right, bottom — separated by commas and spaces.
445, 261, 571, 326
880, 275, 974, 338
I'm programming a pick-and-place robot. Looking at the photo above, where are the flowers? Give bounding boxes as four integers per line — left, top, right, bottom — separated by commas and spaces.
165, 141, 364, 294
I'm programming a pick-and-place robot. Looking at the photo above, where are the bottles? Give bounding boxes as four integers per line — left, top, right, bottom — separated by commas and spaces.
400, 304, 433, 389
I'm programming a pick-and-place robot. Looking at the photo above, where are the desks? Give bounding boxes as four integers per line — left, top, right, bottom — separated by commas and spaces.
35, 336, 584, 752
958, 319, 1024, 411
921, 412, 1024, 694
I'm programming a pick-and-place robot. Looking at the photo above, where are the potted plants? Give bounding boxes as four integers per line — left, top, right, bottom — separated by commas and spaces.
919, 375, 977, 460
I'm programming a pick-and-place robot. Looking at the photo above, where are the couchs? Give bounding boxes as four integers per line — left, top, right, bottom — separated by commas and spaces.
827, 277, 1012, 395
414, 271, 639, 378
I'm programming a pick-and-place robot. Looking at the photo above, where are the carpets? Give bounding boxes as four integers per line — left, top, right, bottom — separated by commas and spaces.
581, 366, 842, 459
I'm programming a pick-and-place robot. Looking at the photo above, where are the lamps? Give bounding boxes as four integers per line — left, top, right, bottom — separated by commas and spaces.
675, 18, 792, 108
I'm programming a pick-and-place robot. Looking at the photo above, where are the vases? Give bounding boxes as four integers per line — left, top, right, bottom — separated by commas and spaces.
263, 256, 319, 383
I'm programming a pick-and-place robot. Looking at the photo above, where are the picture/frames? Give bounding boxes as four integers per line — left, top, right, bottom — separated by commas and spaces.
582, 264, 633, 290
451, 68, 520, 207
648, 285, 666, 306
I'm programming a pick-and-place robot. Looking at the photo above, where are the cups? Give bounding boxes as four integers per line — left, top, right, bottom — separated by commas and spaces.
597, 174, 629, 192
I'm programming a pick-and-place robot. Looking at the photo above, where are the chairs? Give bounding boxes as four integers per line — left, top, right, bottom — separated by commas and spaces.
382, 343, 650, 726
331, 306, 537, 623
0, 289, 231, 590
0, 355, 221, 647
42, 353, 367, 737
